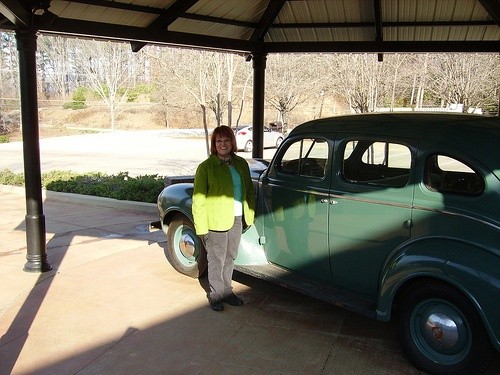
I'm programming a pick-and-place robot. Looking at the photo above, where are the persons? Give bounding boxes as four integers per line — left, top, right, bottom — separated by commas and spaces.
191, 125, 258, 312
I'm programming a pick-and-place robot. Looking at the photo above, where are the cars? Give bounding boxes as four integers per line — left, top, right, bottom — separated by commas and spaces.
149, 112, 500, 375
232, 125, 285, 152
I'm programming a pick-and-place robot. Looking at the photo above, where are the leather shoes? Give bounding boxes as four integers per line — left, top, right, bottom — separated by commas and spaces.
223, 293, 243, 305
212, 299, 224, 311
